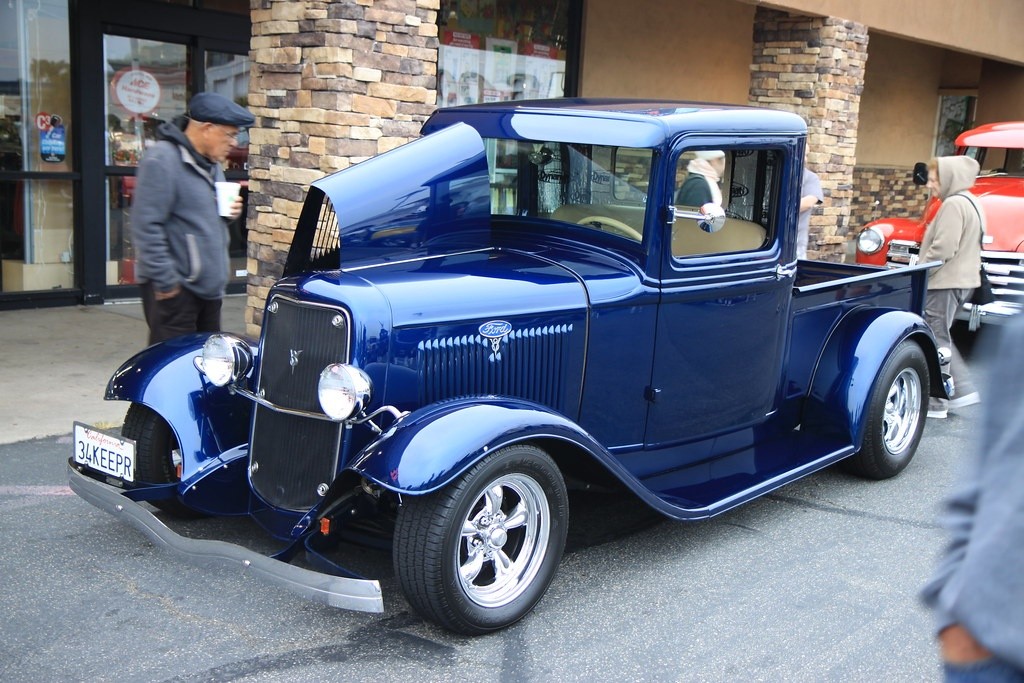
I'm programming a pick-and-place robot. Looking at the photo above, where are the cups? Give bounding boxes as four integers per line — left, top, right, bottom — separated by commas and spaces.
214, 181, 241, 217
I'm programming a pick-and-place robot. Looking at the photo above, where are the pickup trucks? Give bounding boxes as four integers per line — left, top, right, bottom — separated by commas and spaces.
66, 97, 955, 635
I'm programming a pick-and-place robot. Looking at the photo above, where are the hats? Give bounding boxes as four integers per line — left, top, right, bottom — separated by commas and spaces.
695, 150, 725, 160
189, 92, 255, 128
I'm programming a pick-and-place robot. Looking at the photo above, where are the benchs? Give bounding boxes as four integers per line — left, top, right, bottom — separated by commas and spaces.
551, 203, 766, 257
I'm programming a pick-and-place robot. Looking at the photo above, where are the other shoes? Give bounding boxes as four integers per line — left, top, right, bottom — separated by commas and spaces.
947, 392, 981, 409
927, 405, 948, 418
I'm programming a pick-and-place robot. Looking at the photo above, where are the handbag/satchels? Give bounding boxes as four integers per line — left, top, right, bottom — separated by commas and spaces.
969, 264, 996, 306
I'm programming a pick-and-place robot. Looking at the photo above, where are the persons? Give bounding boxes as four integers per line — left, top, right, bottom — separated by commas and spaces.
675, 150, 725, 207
917, 155, 986, 418
130, 92, 256, 345
921, 313, 1024, 683
797, 143, 824, 259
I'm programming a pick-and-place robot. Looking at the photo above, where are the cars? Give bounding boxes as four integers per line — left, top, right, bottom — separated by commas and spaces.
855, 121, 1024, 326
